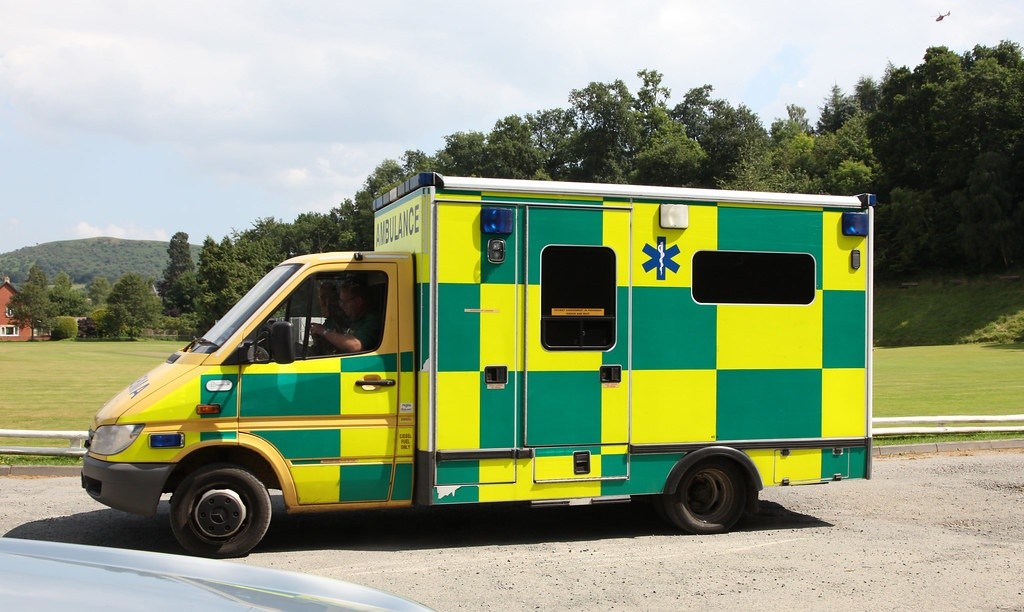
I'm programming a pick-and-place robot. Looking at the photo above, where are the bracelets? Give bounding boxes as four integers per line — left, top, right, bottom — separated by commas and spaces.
321, 328, 332, 337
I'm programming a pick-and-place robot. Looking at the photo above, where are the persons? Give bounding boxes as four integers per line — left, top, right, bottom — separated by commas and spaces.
309, 279, 381, 357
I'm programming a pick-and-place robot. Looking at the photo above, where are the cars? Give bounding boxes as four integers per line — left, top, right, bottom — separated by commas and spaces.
0, 537, 440, 612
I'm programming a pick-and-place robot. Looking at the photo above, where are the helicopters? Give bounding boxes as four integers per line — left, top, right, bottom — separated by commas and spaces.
935, 10, 952, 23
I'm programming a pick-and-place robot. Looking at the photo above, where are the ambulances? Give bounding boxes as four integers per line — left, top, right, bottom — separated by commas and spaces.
80, 167, 875, 561
